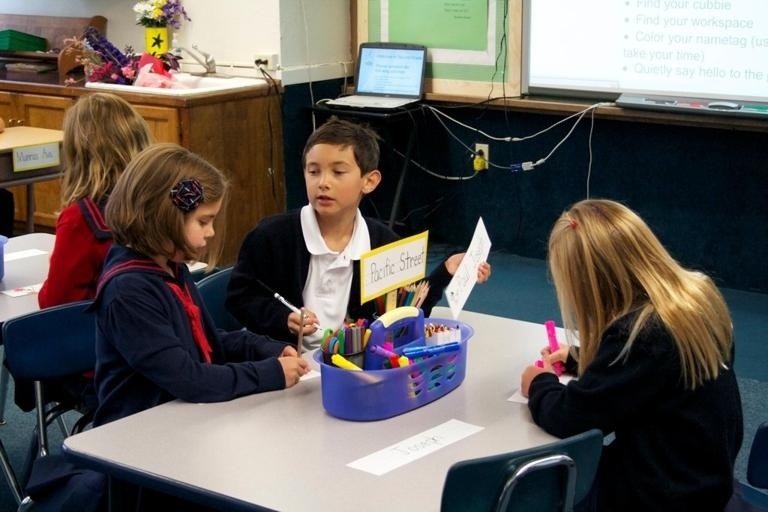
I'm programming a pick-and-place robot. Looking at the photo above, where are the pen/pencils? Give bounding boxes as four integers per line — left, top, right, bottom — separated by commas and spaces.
273, 293, 323, 331
322, 281, 462, 382
545, 320, 562, 377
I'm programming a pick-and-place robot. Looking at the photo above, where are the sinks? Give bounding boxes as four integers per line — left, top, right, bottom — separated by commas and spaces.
84, 74, 268, 96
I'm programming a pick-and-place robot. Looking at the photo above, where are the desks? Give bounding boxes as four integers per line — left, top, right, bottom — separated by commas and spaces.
311, 104, 426, 231
0, 126, 64, 231
61, 306, 579, 512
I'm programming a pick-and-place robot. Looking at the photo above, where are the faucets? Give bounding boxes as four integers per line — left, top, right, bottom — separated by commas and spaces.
177, 44, 217, 73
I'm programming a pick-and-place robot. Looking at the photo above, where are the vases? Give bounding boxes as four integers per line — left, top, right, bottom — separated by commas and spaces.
145, 27, 168, 57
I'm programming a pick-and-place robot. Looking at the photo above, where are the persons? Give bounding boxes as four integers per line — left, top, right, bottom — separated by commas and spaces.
37, 93, 157, 406
88, 143, 311, 427
520, 198, 744, 511
223, 119, 492, 354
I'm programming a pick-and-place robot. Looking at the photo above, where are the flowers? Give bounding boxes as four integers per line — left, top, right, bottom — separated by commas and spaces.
133, 0, 191, 27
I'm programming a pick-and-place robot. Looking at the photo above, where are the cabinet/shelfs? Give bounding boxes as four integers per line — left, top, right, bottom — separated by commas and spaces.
1, 83, 73, 228
74, 86, 284, 271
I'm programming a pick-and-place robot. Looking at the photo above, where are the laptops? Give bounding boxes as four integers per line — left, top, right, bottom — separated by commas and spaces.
325, 42, 427, 111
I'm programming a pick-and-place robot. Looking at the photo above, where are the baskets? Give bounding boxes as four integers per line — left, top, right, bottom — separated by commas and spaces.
312, 318, 475, 424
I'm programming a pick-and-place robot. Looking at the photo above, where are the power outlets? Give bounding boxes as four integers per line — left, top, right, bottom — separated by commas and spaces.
476, 143, 494, 170
254, 53, 277, 71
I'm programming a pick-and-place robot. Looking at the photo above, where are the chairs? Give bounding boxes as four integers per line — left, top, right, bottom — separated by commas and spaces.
724, 421, 768, 512
439, 428, 605, 511
